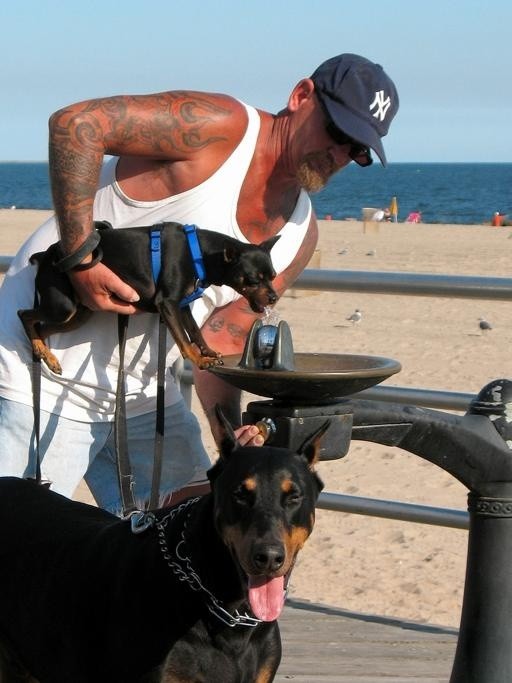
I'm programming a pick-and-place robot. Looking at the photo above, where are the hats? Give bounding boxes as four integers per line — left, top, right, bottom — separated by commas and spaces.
311, 53, 399, 168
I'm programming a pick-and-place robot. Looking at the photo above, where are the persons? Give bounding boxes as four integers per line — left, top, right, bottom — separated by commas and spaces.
492, 211, 503, 225
1, 53, 400, 517
383, 206, 391, 222
405, 209, 423, 223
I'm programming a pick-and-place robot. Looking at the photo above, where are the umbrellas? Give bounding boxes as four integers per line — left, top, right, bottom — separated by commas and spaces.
389, 195, 399, 222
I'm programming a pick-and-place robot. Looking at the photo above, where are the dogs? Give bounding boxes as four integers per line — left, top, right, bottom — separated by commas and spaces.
17, 222, 282, 375
0, 402, 332, 683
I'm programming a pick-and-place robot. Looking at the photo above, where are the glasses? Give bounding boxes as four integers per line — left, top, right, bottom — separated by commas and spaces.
315, 90, 373, 167
